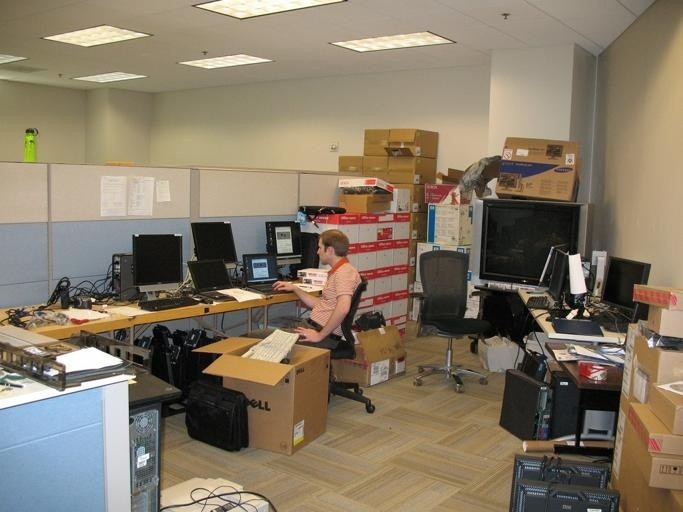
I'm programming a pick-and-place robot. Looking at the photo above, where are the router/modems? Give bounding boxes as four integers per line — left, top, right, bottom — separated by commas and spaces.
59, 281, 69, 309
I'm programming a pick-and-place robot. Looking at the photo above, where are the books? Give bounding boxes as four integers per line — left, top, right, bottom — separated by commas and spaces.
23, 346, 146, 388
544, 342, 624, 365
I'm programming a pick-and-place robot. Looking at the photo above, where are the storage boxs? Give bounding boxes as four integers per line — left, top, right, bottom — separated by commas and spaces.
609, 284, 682, 512
192, 335, 331, 457
496, 137, 582, 201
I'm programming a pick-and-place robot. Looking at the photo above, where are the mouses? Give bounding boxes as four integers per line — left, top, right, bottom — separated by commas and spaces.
202, 298, 213, 304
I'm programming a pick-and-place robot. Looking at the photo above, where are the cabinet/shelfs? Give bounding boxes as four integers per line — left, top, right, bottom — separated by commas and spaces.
0, 336, 135, 512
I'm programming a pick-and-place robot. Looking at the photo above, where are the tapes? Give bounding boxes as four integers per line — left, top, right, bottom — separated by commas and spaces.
586, 365, 608, 381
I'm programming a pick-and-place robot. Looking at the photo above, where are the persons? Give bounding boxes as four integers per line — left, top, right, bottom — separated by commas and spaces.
239, 229, 363, 354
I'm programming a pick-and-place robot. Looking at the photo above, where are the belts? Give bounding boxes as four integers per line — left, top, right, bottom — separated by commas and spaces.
306, 318, 342, 342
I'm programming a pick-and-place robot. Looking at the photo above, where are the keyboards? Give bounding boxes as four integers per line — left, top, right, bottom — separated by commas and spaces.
138, 297, 198, 312
526, 296, 550, 309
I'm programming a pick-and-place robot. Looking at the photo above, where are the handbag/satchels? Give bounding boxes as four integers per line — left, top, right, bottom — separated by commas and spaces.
186, 379, 250, 453
298, 206, 346, 215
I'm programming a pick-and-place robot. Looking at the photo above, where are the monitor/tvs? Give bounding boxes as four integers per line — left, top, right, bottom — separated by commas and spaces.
547, 249, 568, 300
191, 222, 237, 264
471, 198, 588, 291
132, 233, 183, 286
600, 256, 651, 312
266, 220, 303, 259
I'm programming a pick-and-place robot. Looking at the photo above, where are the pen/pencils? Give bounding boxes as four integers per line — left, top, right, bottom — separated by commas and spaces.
0, 382, 22, 387
526, 291, 545, 294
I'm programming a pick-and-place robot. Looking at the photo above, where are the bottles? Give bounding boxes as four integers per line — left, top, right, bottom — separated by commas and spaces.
20, 127, 41, 163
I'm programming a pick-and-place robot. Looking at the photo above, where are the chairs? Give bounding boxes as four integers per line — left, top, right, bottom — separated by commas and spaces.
318, 278, 376, 414
413, 250, 487, 392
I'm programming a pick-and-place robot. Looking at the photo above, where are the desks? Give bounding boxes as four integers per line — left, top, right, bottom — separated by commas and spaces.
468, 281, 533, 356
515, 283, 631, 457
0, 284, 324, 364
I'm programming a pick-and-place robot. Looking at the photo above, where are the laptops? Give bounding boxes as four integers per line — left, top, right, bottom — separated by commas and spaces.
187, 259, 235, 300
243, 253, 281, 292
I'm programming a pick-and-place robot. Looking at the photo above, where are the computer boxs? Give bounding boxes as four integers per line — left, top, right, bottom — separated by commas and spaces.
499, 368, 554, 441
112, 253, 138, 301
129, 402, 161, 512
552, 372, 578, 439
